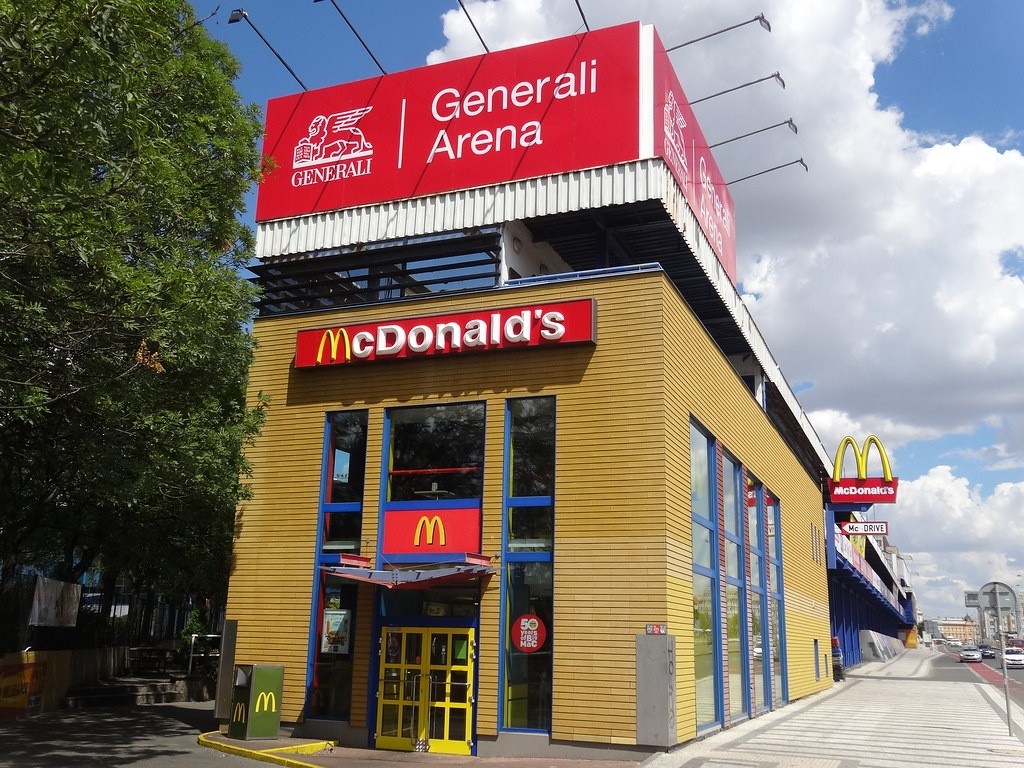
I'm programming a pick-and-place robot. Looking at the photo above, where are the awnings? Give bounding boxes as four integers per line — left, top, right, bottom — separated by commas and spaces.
318, 554, 505, 590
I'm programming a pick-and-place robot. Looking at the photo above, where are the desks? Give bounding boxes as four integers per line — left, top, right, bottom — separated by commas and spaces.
129, 647, 176, 678
413, 490, 458, 501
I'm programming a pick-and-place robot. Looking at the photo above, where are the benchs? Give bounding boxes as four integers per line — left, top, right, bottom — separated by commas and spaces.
124, 657, 173, 679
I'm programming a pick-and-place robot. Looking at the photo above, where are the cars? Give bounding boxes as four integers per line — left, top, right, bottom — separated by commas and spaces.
998, 647, 1024, 669
977, 644, 995, 659
753, 633, 763, 661
944, 636, 968, 647
958, 645, 983, 663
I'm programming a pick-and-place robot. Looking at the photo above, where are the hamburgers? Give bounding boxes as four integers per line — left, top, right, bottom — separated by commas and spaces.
325, 631, 342, 644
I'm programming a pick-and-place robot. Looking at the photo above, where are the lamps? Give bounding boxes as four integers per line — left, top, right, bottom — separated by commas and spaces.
709, 117, 798, 149
726, 157, 809, 185
689, 71, 785, 105
667, 12, 771, 52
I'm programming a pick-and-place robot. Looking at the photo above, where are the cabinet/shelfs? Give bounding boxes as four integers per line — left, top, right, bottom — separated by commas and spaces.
187, 634, 221, 676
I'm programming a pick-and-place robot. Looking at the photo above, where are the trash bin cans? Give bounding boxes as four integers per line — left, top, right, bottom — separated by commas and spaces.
227, 664, 286, 740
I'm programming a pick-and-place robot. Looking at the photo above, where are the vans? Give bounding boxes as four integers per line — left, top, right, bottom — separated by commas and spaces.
1006, 638, 1024, 650
